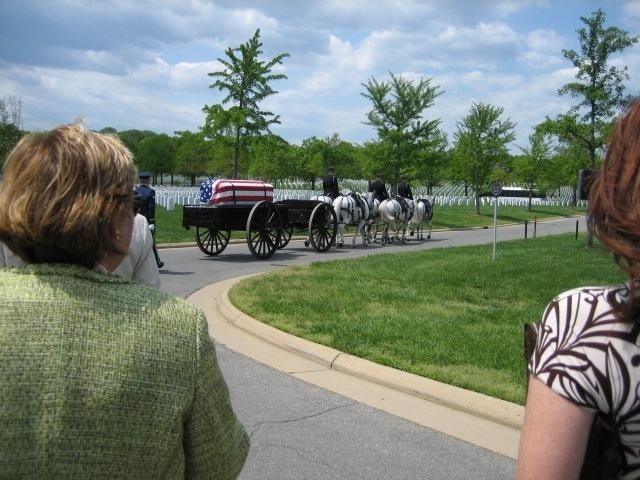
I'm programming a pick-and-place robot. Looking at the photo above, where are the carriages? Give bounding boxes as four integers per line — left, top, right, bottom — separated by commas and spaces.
183, 190, 437, 259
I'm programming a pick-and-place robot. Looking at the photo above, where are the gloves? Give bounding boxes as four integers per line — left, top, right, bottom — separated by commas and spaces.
149, 224, 154, 230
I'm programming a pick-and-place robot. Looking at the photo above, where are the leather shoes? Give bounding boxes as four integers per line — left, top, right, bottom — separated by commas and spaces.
158, 262, 164, 268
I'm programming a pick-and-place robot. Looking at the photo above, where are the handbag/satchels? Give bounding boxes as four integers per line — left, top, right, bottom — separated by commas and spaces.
523, 322, 629, 480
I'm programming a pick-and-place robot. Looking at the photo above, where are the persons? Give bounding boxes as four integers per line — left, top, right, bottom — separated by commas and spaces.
2, 189, 162, 287
514, 101, 640, 478
322, 167, 340, 199
367, 175, 389, 202
398, 176, 413, 200
2, 121, 252, 479
134, 171, 164, 268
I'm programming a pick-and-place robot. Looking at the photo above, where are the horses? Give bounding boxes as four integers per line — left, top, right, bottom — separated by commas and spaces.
409, 198, 433, 240
304, 187, 354, 248
367, 184, 415, 246
331, 189, 376, 249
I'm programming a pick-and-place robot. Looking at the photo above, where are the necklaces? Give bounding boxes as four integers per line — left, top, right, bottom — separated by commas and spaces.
97, 263, 108, 273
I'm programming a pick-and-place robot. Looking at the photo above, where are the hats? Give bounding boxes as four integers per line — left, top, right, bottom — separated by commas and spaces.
138, 172, 151, 179
